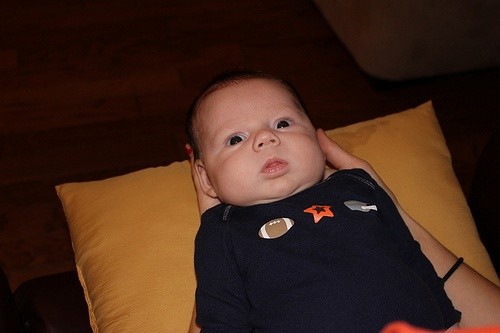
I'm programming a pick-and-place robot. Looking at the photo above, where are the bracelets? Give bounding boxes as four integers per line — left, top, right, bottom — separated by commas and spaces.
440, 256, 464, 284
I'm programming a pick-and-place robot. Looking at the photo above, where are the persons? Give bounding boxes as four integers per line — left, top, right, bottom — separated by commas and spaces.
184, 126, 499, 333
186, 68, 462, 333
